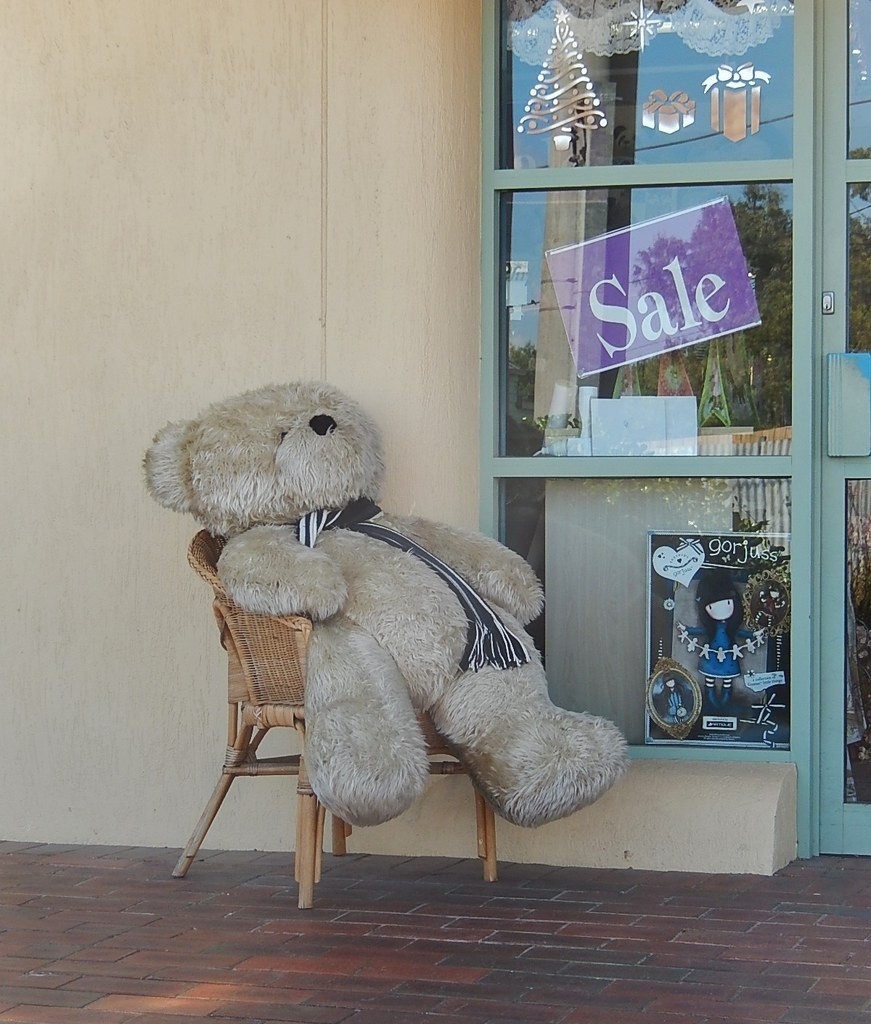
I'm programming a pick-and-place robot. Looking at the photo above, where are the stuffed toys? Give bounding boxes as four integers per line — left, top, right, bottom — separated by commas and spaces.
143, 383, 628, 829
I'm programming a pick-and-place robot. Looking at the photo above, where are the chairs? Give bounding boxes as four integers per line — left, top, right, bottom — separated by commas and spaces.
170, 531, 498, 910
